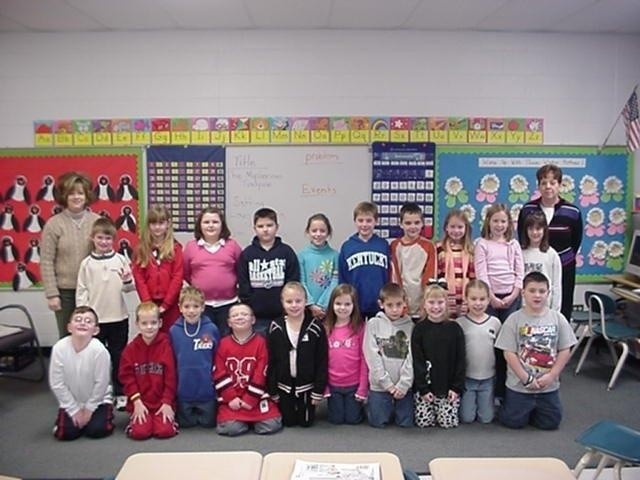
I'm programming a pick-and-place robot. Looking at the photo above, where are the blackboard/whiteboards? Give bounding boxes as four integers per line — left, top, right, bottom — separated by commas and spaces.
221, 143, 374, 259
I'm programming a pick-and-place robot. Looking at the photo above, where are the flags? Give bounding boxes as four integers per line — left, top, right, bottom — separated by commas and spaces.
620, 88, 640, 156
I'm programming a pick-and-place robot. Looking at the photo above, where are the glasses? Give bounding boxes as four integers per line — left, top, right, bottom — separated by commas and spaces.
75, 317, 91, 324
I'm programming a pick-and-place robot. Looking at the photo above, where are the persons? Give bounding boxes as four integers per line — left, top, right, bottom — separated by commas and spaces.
131, 206, 185, 334
75, 219, 135, 412
338, 201, 389, 322
320, 284, 369, 426
411, 284, 465, 429
434, 210, 474, 321
48, 305, 116, 441
517, 164, 583, 324
117, 301, 179, 440
389, 202, 437, 324
520, 210, 563, 313
455, 279, 501, 425
296, 213, 340, 336
237, 207, 300, 335
169, 286, 222, 429
40, 173, 102, 339
362, 282, 416, 429
266, 281, 329, 427
494, 271, 578, 430
212, 304, 283, 437
182, 207, 242, 337
474, 202, 524, 326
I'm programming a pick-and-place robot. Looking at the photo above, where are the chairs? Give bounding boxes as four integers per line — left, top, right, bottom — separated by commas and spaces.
570, 310, 613, 357
0, 303, 46, 383
575, 290, 640, 393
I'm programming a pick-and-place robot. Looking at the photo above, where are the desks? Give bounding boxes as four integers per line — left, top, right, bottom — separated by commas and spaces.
114, 451, 263, 480
603, 275, 640, 305
429, 456, 575, 480
259, 451, 405, 480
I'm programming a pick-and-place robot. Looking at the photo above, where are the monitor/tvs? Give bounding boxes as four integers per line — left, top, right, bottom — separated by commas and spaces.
623, 230, 640, 277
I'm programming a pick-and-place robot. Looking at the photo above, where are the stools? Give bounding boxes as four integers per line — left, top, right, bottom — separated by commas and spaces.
574, 419, 640, 480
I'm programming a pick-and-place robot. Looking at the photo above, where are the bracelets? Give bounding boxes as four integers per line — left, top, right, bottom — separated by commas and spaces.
522, 374, 534, 389
